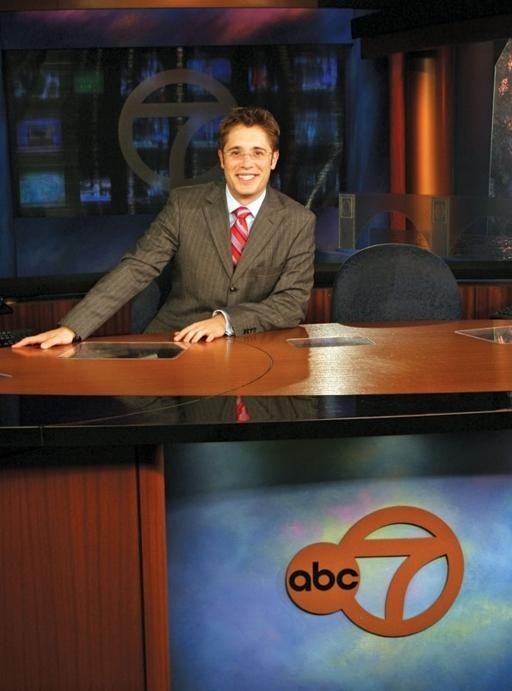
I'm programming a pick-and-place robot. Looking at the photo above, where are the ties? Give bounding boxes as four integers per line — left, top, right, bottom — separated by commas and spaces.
229, 207, 252, 270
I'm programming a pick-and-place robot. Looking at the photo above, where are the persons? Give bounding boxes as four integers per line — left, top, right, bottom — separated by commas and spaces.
7, 104, 320, 350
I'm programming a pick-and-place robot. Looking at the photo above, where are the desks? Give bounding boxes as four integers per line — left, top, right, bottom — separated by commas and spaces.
1, 319, 511, 689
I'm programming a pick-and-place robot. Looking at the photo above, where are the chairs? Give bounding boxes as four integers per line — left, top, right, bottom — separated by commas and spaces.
331, 241, 466, 326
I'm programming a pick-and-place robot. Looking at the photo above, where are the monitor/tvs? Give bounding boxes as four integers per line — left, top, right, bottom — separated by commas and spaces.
69, 166, 115, 212
7, 50, 80, 216
285, 45, 342, 210
112, 47, 184, 217
72, 65, 105, 96
184, 49, 281, 195
